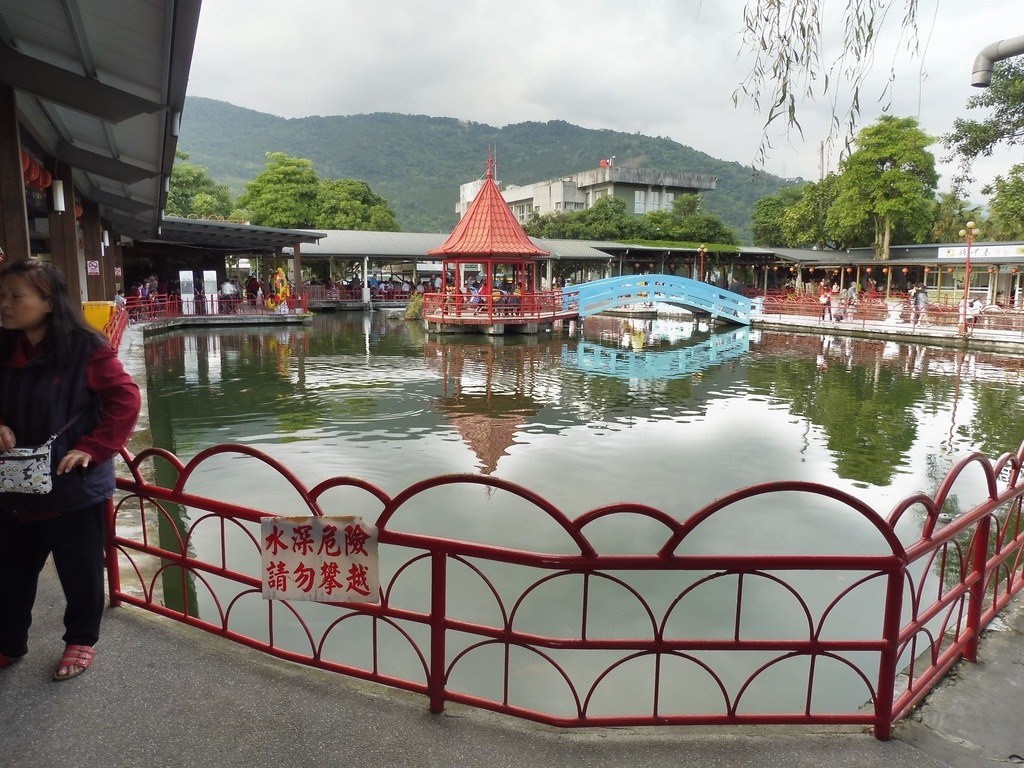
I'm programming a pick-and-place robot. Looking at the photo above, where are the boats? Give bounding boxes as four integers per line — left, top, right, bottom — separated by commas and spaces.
602, 307, 658, 318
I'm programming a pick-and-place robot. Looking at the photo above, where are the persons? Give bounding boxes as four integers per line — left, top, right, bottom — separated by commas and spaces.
816, 277, 1007, 333
116, 290, 130, 327
129, 276, 159, 323
0, 261, 142, 680
220, 268, 576, 315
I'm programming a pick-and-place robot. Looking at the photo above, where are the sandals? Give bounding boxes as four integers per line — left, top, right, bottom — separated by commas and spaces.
0, 654, 23, 669
53, 645, 94, 681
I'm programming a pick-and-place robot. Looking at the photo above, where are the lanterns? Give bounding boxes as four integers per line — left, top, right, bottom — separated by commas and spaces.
610, 262, 1018, 275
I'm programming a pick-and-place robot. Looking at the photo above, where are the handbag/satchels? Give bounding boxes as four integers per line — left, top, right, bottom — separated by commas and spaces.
1, 445, 53, 495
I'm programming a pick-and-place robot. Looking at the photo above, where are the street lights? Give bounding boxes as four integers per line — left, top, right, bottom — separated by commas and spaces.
697, 244, 708, 282
958, 221, 980, 334
